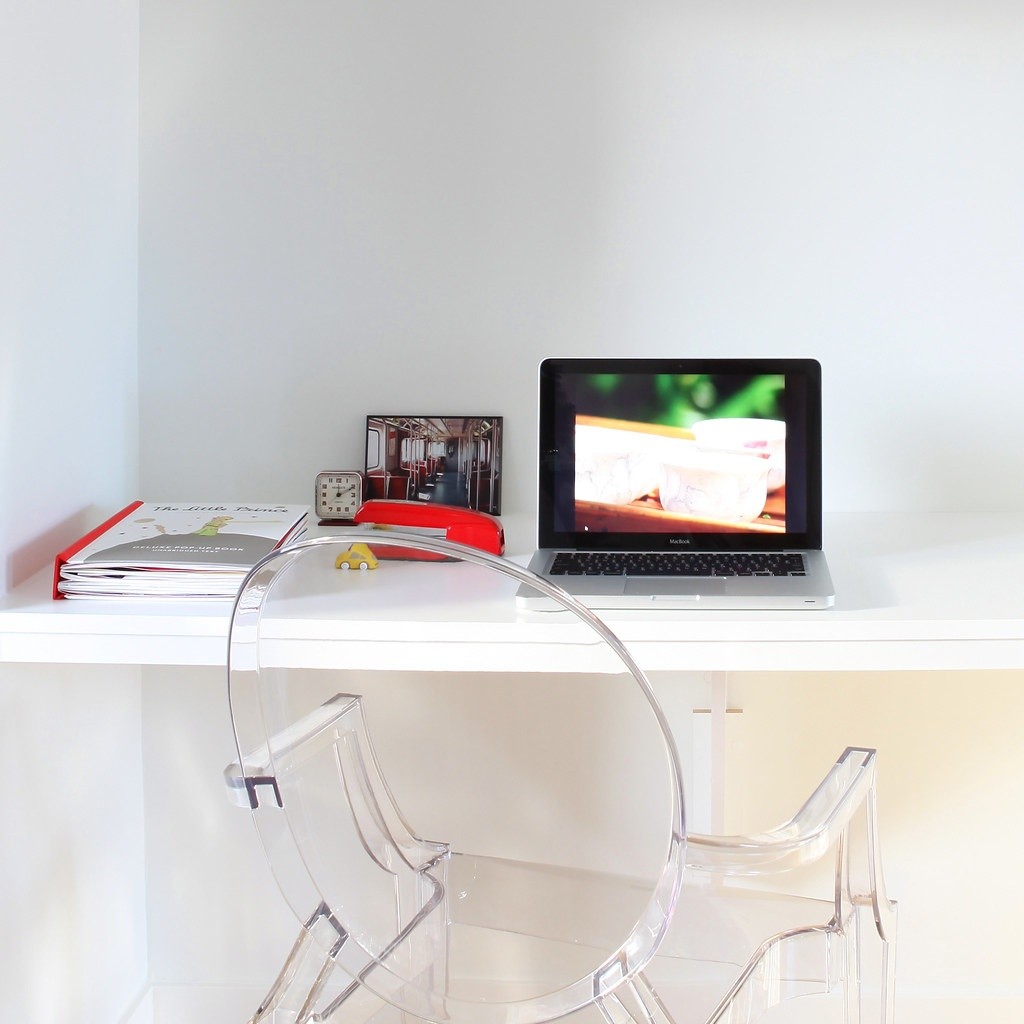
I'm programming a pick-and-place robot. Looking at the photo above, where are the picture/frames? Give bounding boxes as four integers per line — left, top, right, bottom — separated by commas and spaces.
365, 414, 504, 518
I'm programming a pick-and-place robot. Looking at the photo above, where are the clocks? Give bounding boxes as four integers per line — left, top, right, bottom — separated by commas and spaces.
313, 468, 364, 518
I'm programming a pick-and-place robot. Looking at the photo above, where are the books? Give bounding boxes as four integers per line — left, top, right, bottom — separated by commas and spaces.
53, 500, 311, 604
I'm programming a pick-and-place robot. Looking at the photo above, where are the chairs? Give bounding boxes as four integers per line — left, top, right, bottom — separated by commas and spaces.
224, 534, 901, 1024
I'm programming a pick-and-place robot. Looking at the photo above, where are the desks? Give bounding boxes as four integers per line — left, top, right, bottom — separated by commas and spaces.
0, 526, 1022, 676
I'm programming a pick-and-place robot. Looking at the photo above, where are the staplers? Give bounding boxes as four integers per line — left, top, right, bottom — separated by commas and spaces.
355, 500, 506, 563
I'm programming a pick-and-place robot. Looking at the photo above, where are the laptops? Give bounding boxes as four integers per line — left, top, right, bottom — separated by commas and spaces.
515, 358, 835, 611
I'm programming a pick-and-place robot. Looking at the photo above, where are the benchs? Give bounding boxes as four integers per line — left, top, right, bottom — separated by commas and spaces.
369, 455, 439, 501
464, 458, 499, 512
